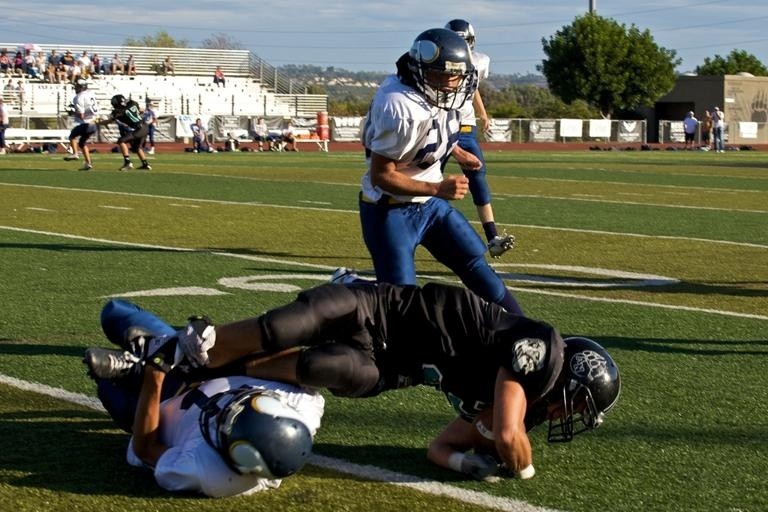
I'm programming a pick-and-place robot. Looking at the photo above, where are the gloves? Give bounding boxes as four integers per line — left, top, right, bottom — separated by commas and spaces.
173, 314, 216, 369
148, 332, 186, 374
463, 453, 504, 484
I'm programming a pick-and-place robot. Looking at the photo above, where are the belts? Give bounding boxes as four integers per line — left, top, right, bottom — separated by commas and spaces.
361, 192, 409, 204
459, 126, 471, 133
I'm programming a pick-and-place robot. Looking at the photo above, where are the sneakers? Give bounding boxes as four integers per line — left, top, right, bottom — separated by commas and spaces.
486, 233, 515, 258
146, 149, 154, 154
330, 266, 359, 283
84, 348, 146, 381
0, 150, 6, 155
62, 154, 154, 172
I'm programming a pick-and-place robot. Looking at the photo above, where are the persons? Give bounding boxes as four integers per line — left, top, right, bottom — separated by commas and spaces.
330, 28, 524, 318
281, 122, 298, 152
81, 282, 621, 484
253, 118, 275, 152
161, 56, 174, 76
215, 66, 225, 88
440, 19, 516, 259
0, 44, 159, 170
97, 300, 325, 499
191, 118, 218, 154
683, 106, 724, 154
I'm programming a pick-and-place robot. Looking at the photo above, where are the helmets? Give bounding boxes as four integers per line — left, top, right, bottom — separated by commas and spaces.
546, 336, 621, 443
110, 94, 126, 109
198, 384, 312, 480
443, 18, 475, 51
73, 78, 87, 93
406, 27, 475, 110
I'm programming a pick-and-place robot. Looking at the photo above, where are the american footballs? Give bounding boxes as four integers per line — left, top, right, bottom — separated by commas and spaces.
473, 406, 494, 453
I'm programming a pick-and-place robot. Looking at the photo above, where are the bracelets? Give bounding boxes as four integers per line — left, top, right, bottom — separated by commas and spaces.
448, 452, 465, 472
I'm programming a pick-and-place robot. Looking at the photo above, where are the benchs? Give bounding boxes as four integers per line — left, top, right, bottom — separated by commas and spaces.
216, 130, 282, 153
4, 128, 74, 156
281, 129, 330, 153
